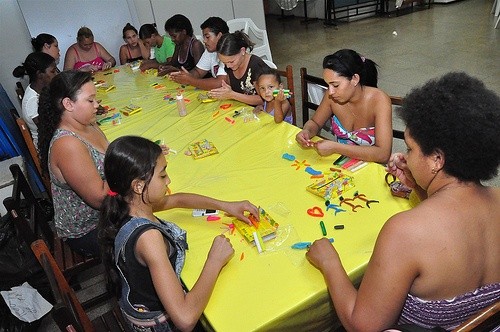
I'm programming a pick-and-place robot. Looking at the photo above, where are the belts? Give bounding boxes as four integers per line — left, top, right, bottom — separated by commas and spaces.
119, 308, 169, 328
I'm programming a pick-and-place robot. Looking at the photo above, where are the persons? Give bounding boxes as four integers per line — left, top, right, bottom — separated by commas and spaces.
305, 72, 500, 332
208, 30, 272, 106
97, 136, 260, 332
120, 23, 150, 65
254, 70, 292, 124
32, 33, 60, 60
139, 24, 176, 72
170, 17, 229, 90
296, 49, 393, 164
13, 51, 98, 156
38, 70, 169, 255
64, 27, 116, 73
158, 14, 210, 79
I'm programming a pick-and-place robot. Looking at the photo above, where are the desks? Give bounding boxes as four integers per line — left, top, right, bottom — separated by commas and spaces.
88, 60, 424, 332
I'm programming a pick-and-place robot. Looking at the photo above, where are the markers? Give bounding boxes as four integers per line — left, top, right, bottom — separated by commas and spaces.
334, 225, 344, 229
320, 221, 327, 235
248, 216, 259, 229
333, 155, 368, 173
225, 117, 234, 124
273, 89, 293, 98
253, 231, 266, 253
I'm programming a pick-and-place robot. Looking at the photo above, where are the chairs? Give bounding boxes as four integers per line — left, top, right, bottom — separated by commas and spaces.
1, 18, 500, 332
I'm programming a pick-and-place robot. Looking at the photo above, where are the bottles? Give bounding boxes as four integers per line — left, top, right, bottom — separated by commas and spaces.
176, 87, 187, 117
